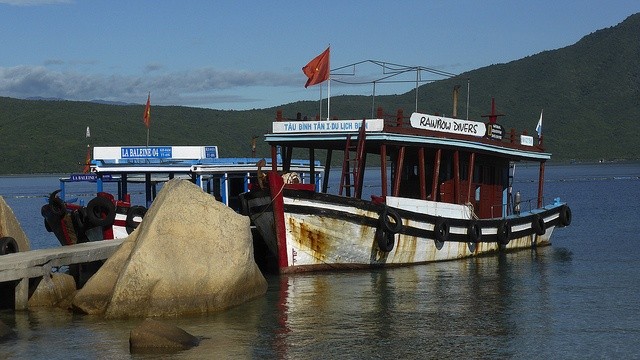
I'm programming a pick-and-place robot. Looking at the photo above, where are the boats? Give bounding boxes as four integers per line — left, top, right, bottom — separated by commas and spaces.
244, 60, 572, 271
42, 146, 324, 261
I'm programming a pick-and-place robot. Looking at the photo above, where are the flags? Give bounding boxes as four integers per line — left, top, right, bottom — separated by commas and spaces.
83, 148, 90, 173
143, 96, 150, 129
302, 46, 330, 89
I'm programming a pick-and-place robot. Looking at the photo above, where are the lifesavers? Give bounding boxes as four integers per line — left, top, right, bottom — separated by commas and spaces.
127, 205, 147, 229
0, 237, 19, 255
434, 218, 450, 242
468, 221, 482, 243
532, 214, 545, 235
497, 219, 511, 244
87, 197, 116, 227
379, 208, 402, 234
560, 206, 571, 226
378, 228, 394, 252
71, 207, 87, 230
49, 198, 65, 217
